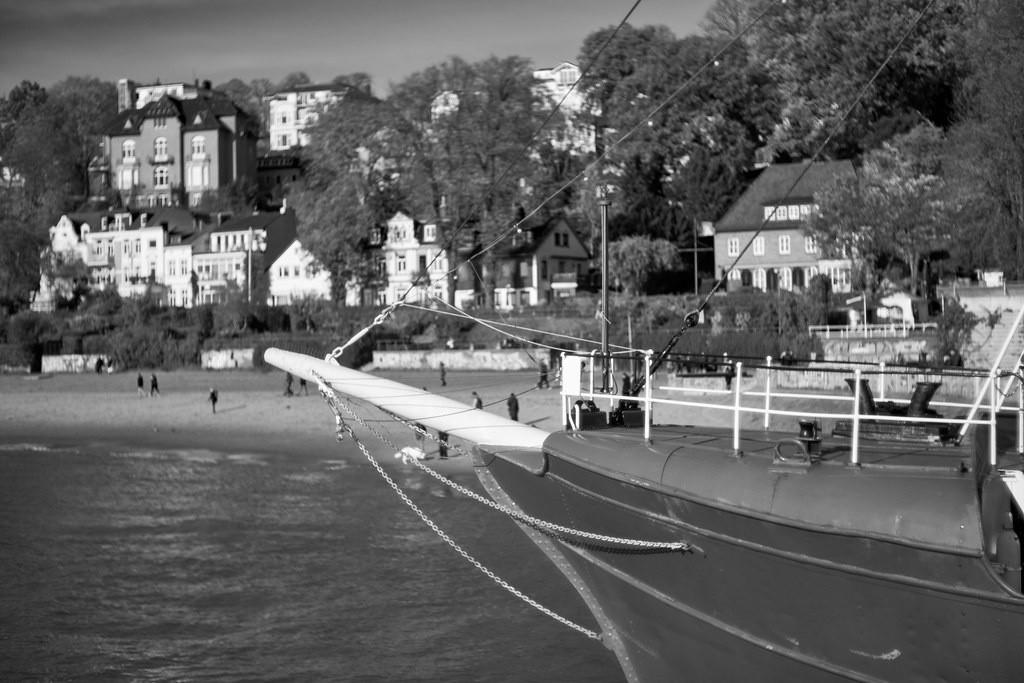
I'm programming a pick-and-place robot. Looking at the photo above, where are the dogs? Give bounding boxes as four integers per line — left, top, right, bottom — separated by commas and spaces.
394, 446, 426, 464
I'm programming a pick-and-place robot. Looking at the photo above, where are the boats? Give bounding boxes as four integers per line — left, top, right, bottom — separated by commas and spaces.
263, 0, 1024, 683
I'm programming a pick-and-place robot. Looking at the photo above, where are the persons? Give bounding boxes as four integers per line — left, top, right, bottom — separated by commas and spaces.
622, 372, 640, 396
94, 353, 114, 375
335, 387, 520, 460
136, 372, 161, 399
282, 372, 309, 397
446, 335, 455, 349
673, 347, 794, 378
207, 385, 218, 414
438, 362, 447, 386
537, 357, 551, 390
